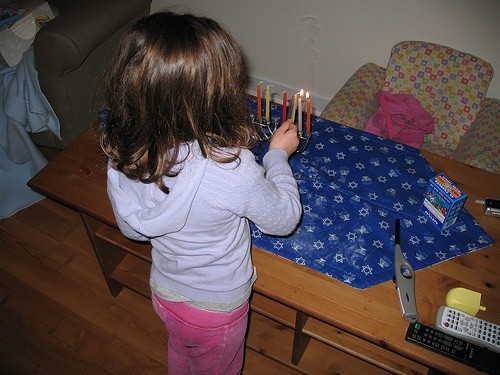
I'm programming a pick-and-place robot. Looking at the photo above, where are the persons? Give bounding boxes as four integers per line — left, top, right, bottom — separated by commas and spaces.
94, 10, 302, 375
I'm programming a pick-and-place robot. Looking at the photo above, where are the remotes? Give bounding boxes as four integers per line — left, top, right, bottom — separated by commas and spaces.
435, 306, 500, 355
406, 322, 500, 375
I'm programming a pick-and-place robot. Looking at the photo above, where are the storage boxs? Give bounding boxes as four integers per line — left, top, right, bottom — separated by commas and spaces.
419, 173, 468, 232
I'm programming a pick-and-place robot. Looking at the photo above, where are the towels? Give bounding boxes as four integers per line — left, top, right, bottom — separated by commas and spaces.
0, 42, 64, 221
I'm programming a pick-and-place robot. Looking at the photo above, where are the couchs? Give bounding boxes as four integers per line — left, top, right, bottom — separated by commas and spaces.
0, 0, 152, 150
320, 41, 500, 175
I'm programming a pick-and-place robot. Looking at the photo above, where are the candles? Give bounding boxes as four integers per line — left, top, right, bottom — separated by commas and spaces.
256, 82, 311, 134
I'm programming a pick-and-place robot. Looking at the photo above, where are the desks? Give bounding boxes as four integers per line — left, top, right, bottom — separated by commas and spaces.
26, 96, 500, 375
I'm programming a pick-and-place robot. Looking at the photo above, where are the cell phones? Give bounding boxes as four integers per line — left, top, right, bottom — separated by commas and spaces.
475, 198, 500, 217
